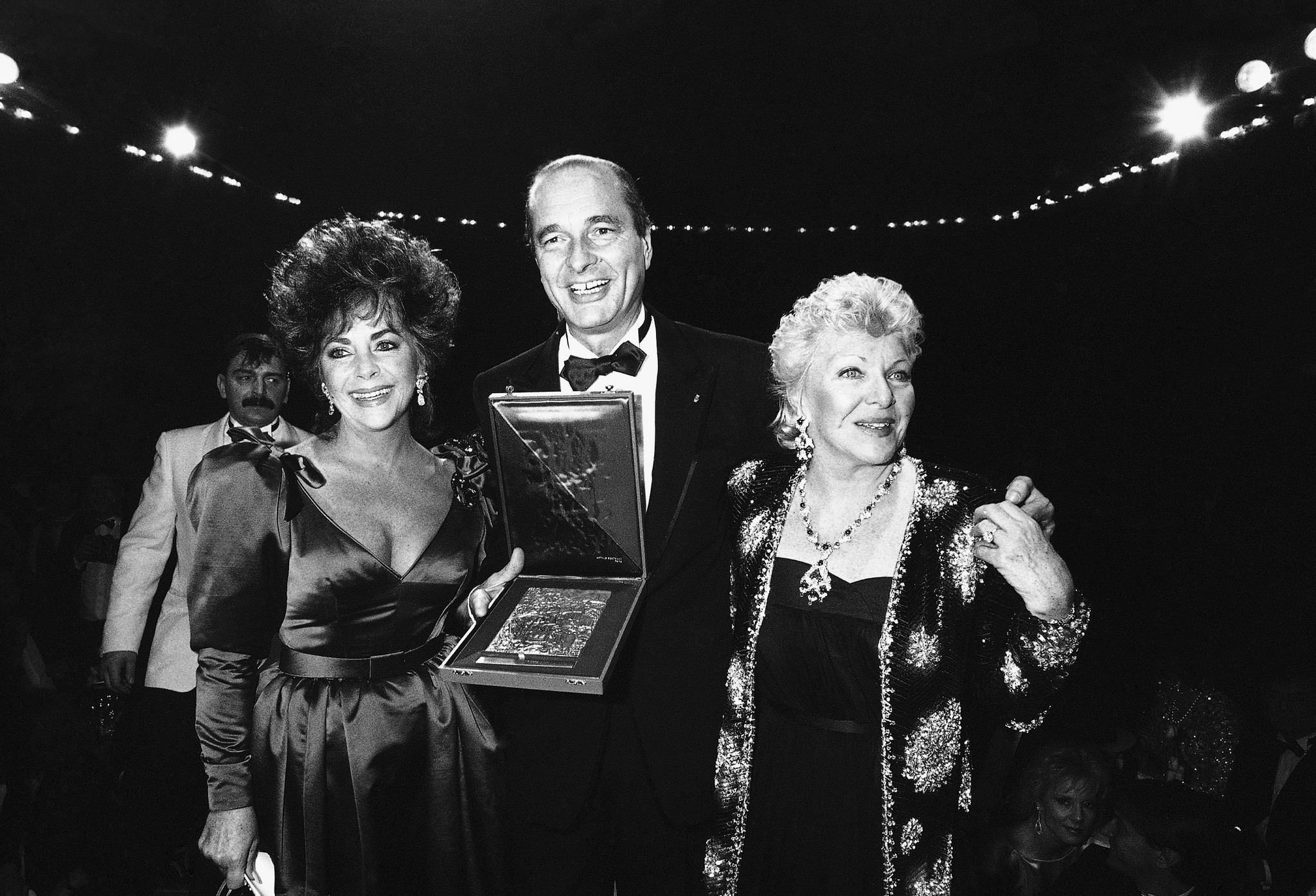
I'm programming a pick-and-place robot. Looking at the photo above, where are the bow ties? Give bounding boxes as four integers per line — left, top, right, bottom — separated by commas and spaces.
558, 340, 647, 392
227, 426, 276, 443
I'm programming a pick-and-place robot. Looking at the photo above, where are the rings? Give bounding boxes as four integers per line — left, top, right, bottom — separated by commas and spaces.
981, 527, 998, 542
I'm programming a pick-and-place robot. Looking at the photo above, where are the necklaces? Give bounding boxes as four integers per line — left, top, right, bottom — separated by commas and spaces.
797, 456, 901, 605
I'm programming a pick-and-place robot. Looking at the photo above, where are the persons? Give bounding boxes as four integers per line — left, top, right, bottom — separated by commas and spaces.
699, 270, 1094, 896
182, 217, 524, 896
94, 330, 316, 895
462, 153, 788, 896
